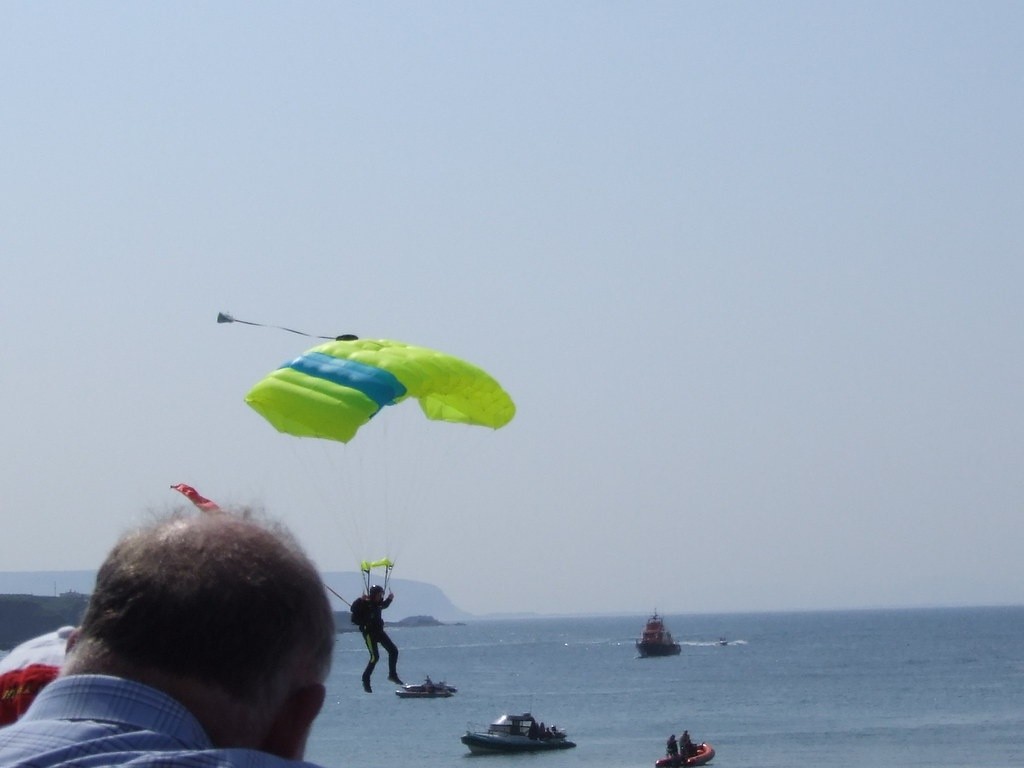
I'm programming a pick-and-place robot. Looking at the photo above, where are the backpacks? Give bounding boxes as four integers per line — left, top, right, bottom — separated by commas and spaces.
350, 595, 370, 625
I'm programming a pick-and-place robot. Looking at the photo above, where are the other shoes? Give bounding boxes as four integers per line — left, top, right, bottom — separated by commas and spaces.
362, 672, 373, 693
387, 673, 404, 685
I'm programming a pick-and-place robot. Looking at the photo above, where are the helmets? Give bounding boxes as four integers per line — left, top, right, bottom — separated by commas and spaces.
370, 585, 385, 597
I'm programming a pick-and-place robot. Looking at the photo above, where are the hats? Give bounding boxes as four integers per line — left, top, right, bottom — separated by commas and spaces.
0, 626, 75, 728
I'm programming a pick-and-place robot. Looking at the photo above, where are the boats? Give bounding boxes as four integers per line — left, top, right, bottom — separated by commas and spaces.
655, 743, 715, 768
719, 638, 727, 646
462, 712, 577, 755
635, 614, 681, 656
395, 683, 457, 698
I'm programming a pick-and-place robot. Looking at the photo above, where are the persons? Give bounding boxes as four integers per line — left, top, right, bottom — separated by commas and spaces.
666, 730, 695, 762
351, 585, 404, 692
529, 723, 555, 741
0, 504, 337, 768
